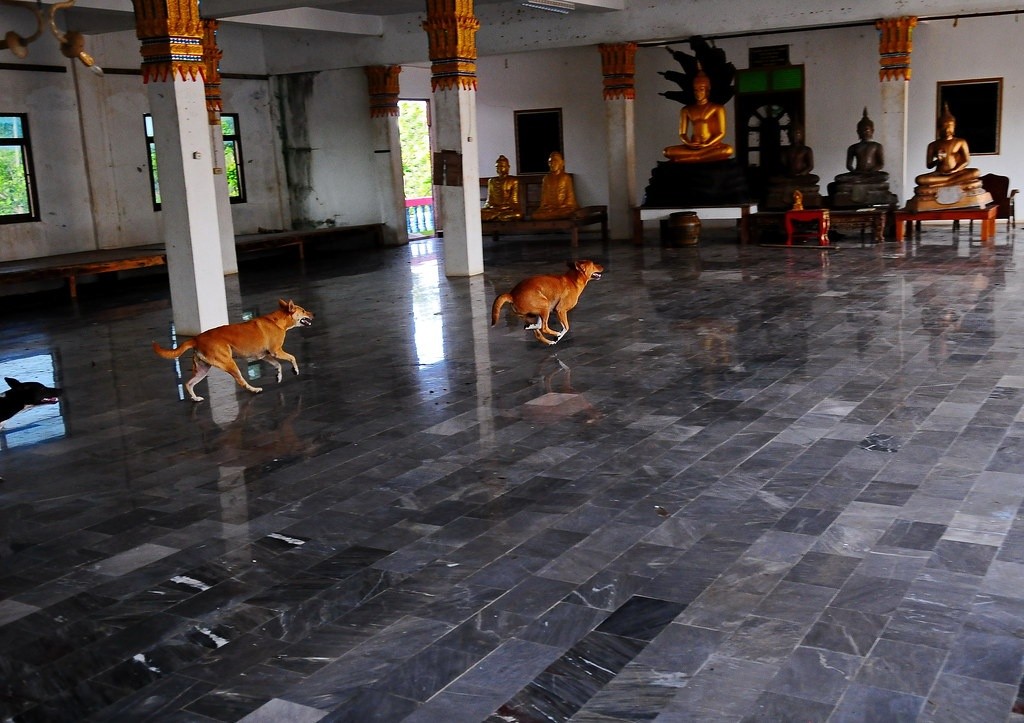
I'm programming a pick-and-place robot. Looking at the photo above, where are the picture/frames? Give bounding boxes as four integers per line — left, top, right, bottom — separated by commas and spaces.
936, 76, 1004, 156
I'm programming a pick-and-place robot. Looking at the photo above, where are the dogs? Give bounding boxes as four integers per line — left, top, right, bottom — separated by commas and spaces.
490, 257, 604, 346
152, 298, 313, 403
0, 377, 63, 424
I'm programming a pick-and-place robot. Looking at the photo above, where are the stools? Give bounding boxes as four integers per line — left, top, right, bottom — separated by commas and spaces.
785, 208, 831, 246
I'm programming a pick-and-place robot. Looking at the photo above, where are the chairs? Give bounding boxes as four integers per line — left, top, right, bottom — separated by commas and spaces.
970, 173, 1015, 226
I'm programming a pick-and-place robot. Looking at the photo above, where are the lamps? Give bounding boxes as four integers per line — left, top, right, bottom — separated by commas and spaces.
0, 0, 105, 78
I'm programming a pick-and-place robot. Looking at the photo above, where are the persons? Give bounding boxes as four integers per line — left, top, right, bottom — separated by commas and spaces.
530, 150, 579, 220
662, 70, 735, 163
915, 101, 979, 187
481, 155, 526, 222
834, 107, 890, 184
770, 111, 820, 186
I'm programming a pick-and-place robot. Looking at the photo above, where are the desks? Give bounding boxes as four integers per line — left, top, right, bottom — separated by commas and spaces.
893, 204, 1000, 242
123, 222, 385, 262
0, 249, 166, 297
750, 205, 899, 244
480, 172, 574, 202
630, 203, 758, 250
482, 205, 609, 248
